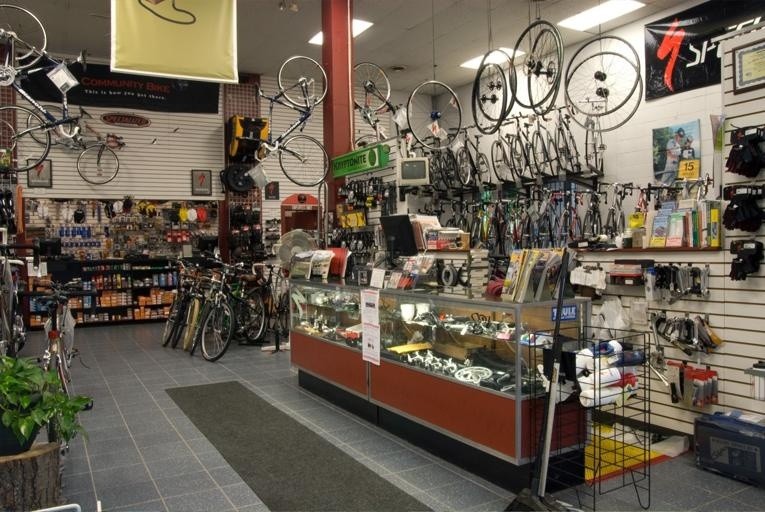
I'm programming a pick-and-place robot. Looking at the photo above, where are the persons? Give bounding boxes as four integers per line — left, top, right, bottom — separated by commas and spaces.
679, 135, 695, 160
660, 128, 686, 185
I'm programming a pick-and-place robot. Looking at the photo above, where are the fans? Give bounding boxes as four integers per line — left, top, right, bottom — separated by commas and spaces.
274, 229, 315, 264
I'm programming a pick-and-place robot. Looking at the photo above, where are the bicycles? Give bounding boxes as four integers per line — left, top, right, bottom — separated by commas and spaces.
397, 181, 685, 246
2, 3, 92, 176
345, 60, 462, 162
27, 101, 122, 185
0, 240, 42, 357
235, 56, 331, 189
373, 96, 608, 181
160, 256, 292, 363
22, 286, 102, 410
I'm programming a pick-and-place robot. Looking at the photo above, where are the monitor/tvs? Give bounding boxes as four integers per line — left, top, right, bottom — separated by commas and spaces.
379, 215, 418, 270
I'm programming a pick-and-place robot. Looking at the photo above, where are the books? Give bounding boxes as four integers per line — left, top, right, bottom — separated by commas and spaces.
627, 199, 720, 247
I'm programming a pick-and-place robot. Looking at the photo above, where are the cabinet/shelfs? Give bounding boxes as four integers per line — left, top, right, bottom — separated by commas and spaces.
288, 280, 378, 444
369, 287, 591, 500
24, 255, 184, 329
